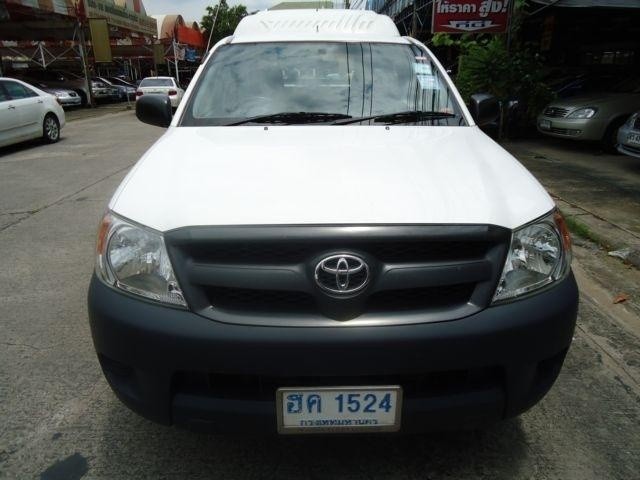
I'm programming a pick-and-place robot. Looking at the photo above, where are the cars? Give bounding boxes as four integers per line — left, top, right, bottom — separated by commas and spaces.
83, 7, 579, 448
442, 55, 639, 163
0, 68, 192, 152
